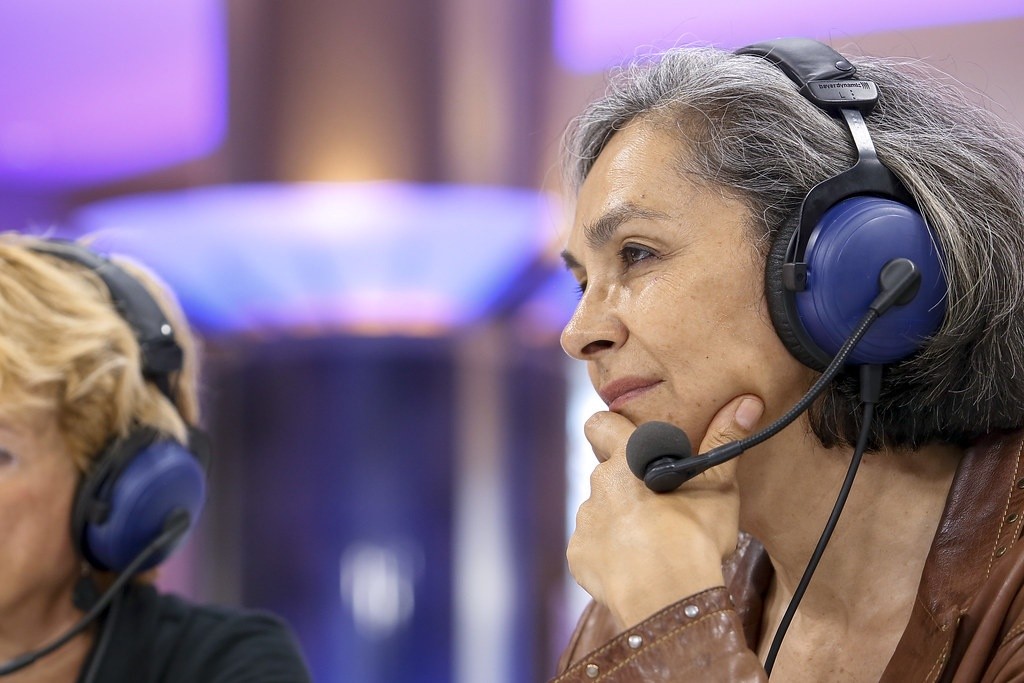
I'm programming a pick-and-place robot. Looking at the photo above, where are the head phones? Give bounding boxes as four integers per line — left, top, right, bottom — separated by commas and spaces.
25, 238, 206, 575
733, 36, 954, 374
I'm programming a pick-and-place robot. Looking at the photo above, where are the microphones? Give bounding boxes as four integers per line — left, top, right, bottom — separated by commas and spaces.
625, 259, 920, 494
0, 507, 190, 679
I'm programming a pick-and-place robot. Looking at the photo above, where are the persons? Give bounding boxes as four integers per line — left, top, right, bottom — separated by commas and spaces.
0, 233, 311, 683
544, 40, 1024, 683
